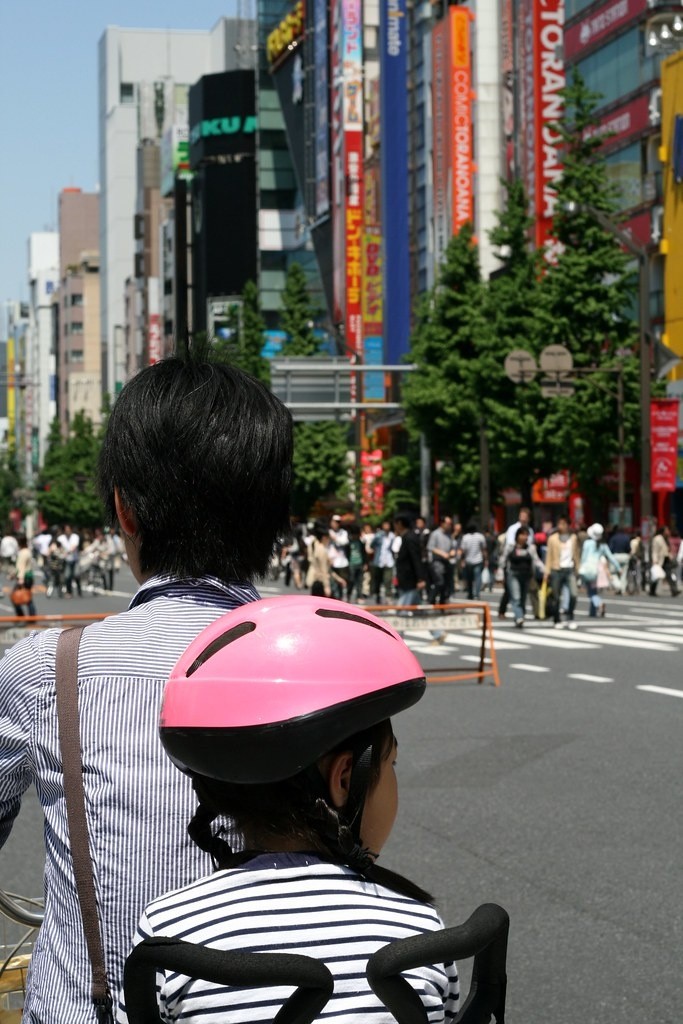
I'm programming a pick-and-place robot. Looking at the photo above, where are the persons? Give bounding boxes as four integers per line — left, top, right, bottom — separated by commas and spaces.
116, 596, 460, 1024
7, 534, 37, 627
0, 532, 19, 565
270, 506, 683, 630
0, 355, 294, 1024
32, 525, 129, 600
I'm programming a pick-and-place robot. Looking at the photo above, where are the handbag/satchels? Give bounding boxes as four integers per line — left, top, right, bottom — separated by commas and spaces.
650, 564, 666, 581
12, 583, 31, 605
538, 580, 552, 619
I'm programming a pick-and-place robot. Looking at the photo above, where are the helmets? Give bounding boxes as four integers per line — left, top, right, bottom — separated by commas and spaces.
158, 594, 427, 783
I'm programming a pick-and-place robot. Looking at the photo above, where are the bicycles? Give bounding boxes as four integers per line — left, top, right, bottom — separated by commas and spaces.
0, 891, 511, 1024
41, 551, 70, 598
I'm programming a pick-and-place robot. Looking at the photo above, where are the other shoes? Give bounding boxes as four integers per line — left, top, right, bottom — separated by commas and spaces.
516, 618, 523, 629
599, 602, 606, 619
671, 590, 681, 597
498, 612, 506, 620
649, 591, 657, 596
432, 631, 448, 646
554, 622, 566, 629
567, 620, 578, 630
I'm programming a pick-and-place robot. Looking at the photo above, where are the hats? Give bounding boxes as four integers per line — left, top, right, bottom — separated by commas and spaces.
587, 522, 604, 540
